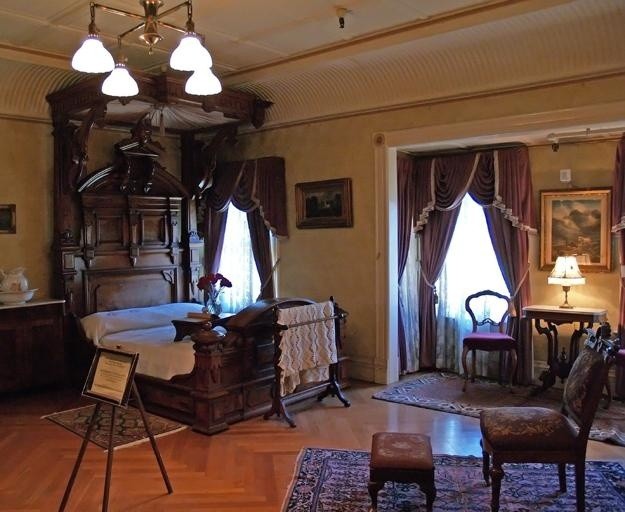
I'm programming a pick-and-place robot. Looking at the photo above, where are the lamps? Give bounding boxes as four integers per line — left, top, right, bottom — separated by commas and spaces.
71, 0, 221, 96
548, 255, 586, 308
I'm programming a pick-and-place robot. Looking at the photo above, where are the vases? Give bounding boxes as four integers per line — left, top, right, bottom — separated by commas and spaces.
206, 292, 222, 315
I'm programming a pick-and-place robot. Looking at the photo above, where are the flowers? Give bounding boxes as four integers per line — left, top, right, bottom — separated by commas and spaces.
197, 273, 233, 312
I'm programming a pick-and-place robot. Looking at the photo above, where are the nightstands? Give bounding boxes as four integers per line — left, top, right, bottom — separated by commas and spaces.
0, 299, 66, 395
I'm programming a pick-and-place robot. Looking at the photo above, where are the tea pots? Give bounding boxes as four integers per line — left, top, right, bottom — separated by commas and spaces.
0, 267, 29, 291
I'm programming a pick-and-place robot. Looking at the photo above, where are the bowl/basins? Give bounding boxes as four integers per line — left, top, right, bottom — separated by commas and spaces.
1, 288, 38, 306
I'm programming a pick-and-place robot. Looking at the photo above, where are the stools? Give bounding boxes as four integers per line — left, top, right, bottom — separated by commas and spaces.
369, 432, 437, 512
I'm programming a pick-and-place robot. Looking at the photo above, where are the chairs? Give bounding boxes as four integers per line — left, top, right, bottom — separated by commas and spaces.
480, 322, 621, 512
603, 350, 625, 409
462, 290, 518, 392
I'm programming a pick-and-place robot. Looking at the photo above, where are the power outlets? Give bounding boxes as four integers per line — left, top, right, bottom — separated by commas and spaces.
560, 169, 571, 181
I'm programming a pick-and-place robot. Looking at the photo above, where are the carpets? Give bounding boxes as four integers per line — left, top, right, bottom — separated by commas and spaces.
372, 372, 625, 446
41, 402, 188, 452
280, 447, 625, 512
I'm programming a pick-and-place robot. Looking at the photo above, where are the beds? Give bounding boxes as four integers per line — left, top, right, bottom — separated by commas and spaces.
46, 67, 352, 436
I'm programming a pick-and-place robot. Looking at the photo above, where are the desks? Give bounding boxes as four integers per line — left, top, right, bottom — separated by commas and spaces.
522, 305, 606, 396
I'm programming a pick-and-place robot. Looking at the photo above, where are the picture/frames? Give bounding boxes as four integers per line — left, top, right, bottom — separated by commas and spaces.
294, 178, 354, 228
539, 190, 611, 272
0, 204, 16, 234
